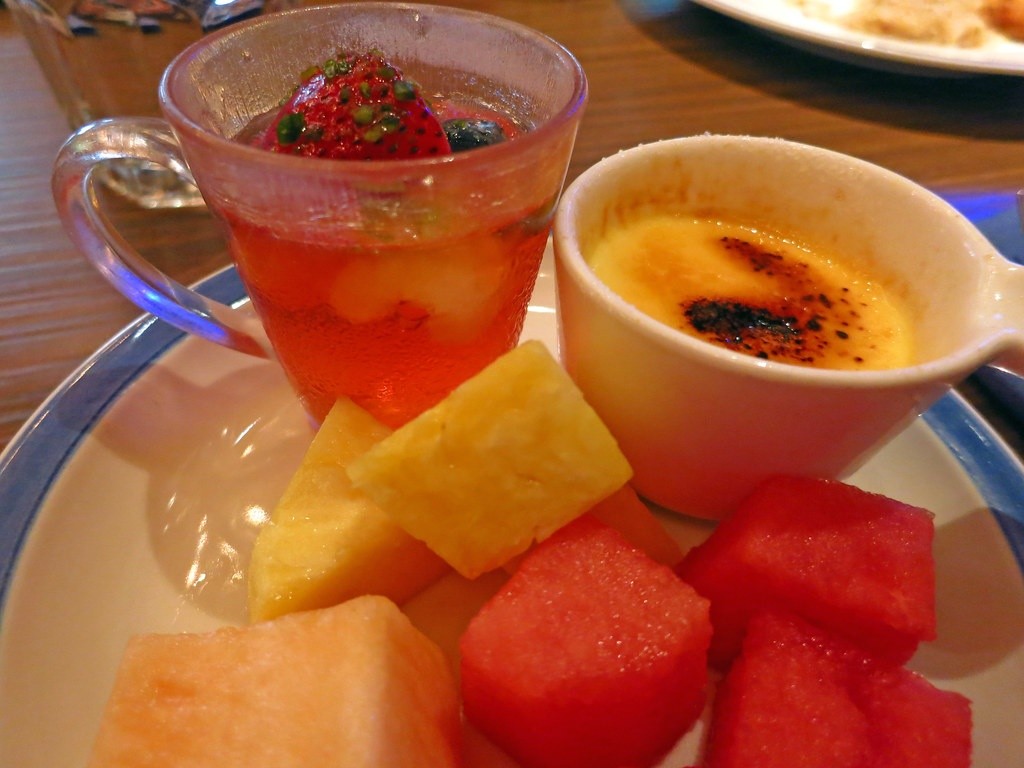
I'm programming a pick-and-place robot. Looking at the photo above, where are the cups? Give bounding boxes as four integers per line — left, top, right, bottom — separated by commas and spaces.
552, 132, 1024, 520
6, 0, 300, 211
50, 3, 587, 428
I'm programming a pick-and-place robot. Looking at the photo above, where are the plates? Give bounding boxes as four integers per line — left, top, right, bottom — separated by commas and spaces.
694, 0, 1024, 74
926, 185, 1024, 423
2, 234, 1024, 767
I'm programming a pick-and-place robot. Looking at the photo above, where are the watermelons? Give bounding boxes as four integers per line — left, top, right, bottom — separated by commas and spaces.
460, 473, 975, 768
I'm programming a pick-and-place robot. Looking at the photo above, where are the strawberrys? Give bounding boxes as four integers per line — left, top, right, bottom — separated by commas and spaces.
236, 54, 451, 231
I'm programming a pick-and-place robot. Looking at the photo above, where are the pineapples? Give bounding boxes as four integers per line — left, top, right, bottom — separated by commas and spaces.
247, 340, 633, 623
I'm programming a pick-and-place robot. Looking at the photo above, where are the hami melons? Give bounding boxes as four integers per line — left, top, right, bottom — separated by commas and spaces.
91, 594, 458, 768
